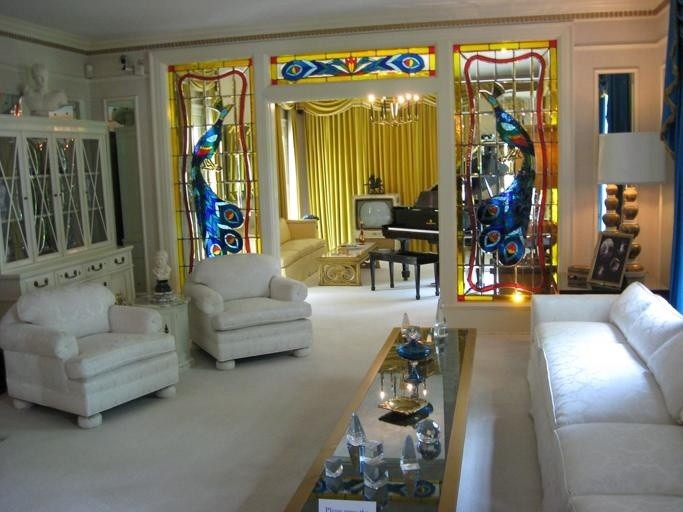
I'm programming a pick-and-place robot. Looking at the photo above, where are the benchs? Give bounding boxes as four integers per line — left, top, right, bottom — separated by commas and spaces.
370, 248, 439, 300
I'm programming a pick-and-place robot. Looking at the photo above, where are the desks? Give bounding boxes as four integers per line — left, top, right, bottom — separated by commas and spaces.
557, 270, 669, 300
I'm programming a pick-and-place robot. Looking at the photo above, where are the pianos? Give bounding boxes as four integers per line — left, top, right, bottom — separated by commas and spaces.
382, 157, 509, 289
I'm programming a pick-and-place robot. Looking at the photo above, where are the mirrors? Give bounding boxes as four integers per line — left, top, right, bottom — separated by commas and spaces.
100, 95, 150, 298
594, 67, 637, 236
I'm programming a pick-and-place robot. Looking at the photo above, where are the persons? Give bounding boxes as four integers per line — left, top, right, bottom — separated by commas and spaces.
152, 251, 170, 281
591, 237, 629, 283
27, 63, 67, 113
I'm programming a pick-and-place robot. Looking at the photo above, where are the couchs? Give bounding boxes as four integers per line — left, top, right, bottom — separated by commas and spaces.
0, 282, 180, 430
525, 282, 682, 512
181, 252, 313, 371
277, 217, 325, 281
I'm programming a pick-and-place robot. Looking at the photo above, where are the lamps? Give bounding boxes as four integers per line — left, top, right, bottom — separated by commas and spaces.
596, 132, 667, 279
367, 91, 420, 127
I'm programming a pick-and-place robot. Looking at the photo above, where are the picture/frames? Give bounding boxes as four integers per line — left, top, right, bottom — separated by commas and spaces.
588, 232, 634, 289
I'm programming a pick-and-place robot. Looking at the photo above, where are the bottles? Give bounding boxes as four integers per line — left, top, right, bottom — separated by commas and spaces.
432, 300, 449, 339
359, 223, 365, 243
433, 340, 448, 374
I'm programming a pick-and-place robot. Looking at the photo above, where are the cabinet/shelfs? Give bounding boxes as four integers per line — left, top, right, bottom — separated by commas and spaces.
0, 244, 135, 326
0, 114, 114, 269
134, 296, 194, 371
